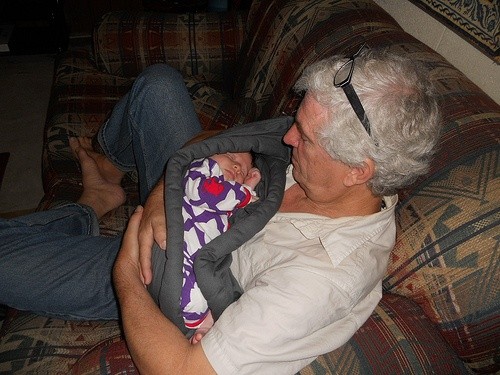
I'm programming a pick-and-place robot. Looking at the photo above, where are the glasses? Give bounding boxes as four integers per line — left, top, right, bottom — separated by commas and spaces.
333, 40, 379, 148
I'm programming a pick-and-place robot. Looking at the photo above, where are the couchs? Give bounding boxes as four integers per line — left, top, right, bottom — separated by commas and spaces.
1, 0, 499, 375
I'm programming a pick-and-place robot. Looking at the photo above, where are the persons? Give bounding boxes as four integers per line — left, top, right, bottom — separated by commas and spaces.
140, 149, 263, 349
0, 42, 444, 375
